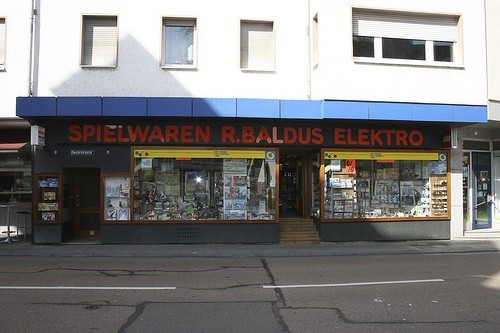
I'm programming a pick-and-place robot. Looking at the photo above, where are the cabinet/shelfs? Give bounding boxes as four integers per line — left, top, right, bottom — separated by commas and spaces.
319, 148, 451, 220
32, 172, 62, 225
130, 146, 280, 224
310, 152, 320, 219
100, 171, 131, 225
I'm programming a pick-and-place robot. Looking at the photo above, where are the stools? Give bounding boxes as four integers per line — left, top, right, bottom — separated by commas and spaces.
16, 211, 32, 240
